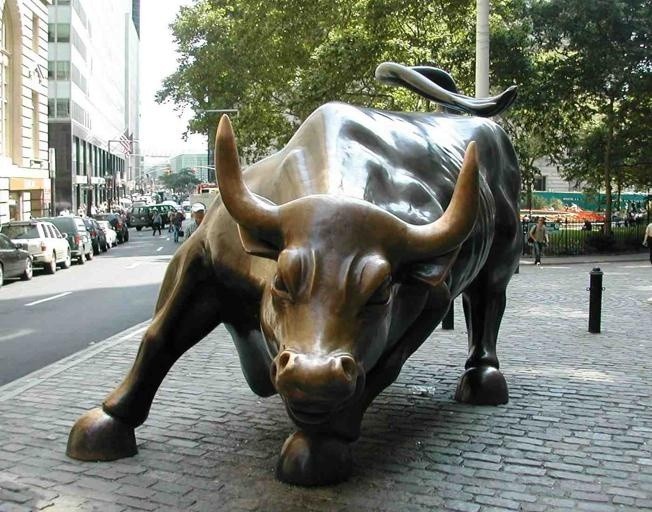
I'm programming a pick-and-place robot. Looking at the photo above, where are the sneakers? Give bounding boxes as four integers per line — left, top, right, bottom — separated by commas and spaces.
174, 240, 178, 243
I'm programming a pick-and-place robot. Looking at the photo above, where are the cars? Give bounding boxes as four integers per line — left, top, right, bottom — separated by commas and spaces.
0, 183, 217, 285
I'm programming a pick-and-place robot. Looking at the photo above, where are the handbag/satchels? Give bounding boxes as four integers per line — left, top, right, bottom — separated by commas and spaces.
528, 235, 535, 244
178, 229, 184, 237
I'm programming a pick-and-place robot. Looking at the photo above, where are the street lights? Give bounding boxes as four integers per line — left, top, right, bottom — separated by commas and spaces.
197, 108, 240, 120
106, 138, 140, 213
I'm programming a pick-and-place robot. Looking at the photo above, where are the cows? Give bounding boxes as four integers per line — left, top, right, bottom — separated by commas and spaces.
66, 60, 524, 489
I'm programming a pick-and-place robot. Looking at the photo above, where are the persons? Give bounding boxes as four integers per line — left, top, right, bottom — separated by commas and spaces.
150, 209, 185, 244
182, 202, 208, 242
641, 222, 652, 267
527, 216, 549, 267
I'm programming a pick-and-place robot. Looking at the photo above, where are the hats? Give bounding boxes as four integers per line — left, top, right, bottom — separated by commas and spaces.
191, 202, 205, 213
